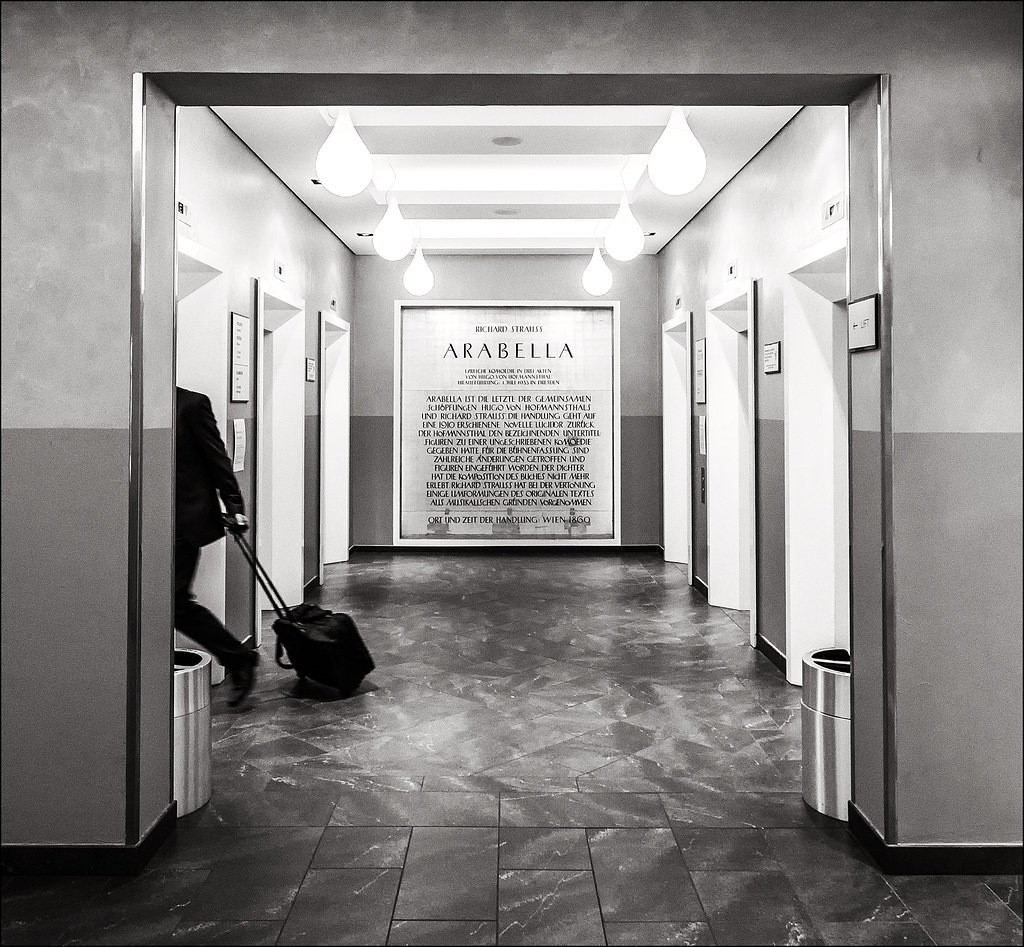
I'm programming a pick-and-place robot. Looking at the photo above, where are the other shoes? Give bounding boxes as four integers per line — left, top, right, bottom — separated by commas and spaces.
225, 651, 260, 707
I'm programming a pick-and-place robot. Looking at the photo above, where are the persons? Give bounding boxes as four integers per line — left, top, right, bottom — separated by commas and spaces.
175, 385, 260, 707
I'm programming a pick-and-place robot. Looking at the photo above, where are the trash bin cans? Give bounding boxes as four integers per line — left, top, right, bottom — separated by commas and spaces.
801, 647, 851, 827
171, 648, 213, 821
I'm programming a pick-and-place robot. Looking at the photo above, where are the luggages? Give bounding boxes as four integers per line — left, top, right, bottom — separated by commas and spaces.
228, 525, 374, 702
